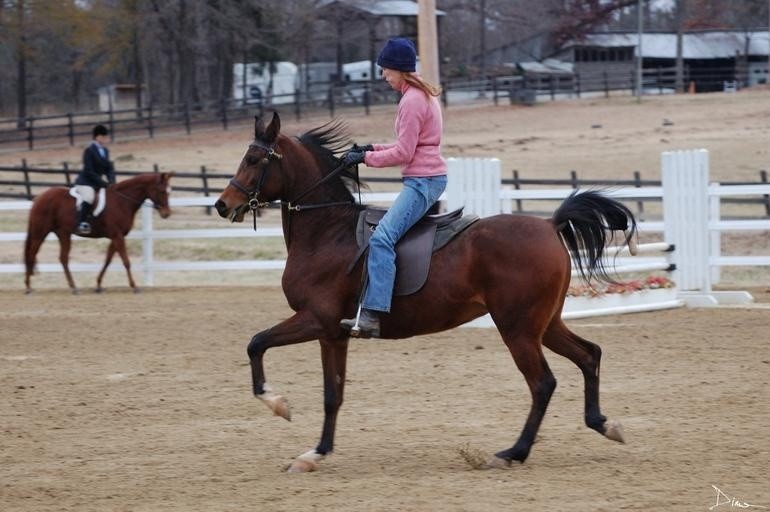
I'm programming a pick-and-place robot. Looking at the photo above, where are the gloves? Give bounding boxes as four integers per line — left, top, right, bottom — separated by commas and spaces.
340, 144, 372, 166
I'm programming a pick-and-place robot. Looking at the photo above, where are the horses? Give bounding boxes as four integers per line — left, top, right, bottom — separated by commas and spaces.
214, 107, 640, 474
23, 169, 175, 295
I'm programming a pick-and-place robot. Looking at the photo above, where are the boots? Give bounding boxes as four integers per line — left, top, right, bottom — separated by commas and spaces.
339, 309, 380, 337
70, 200, 92, 233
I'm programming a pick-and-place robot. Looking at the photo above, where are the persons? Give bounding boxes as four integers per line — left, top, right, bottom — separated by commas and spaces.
74, 125, 116, 234
336, 33, 450, 341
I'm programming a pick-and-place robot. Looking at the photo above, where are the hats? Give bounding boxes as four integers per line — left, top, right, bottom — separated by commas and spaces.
94, 125, 107, 135
377, 39, 417, 72
